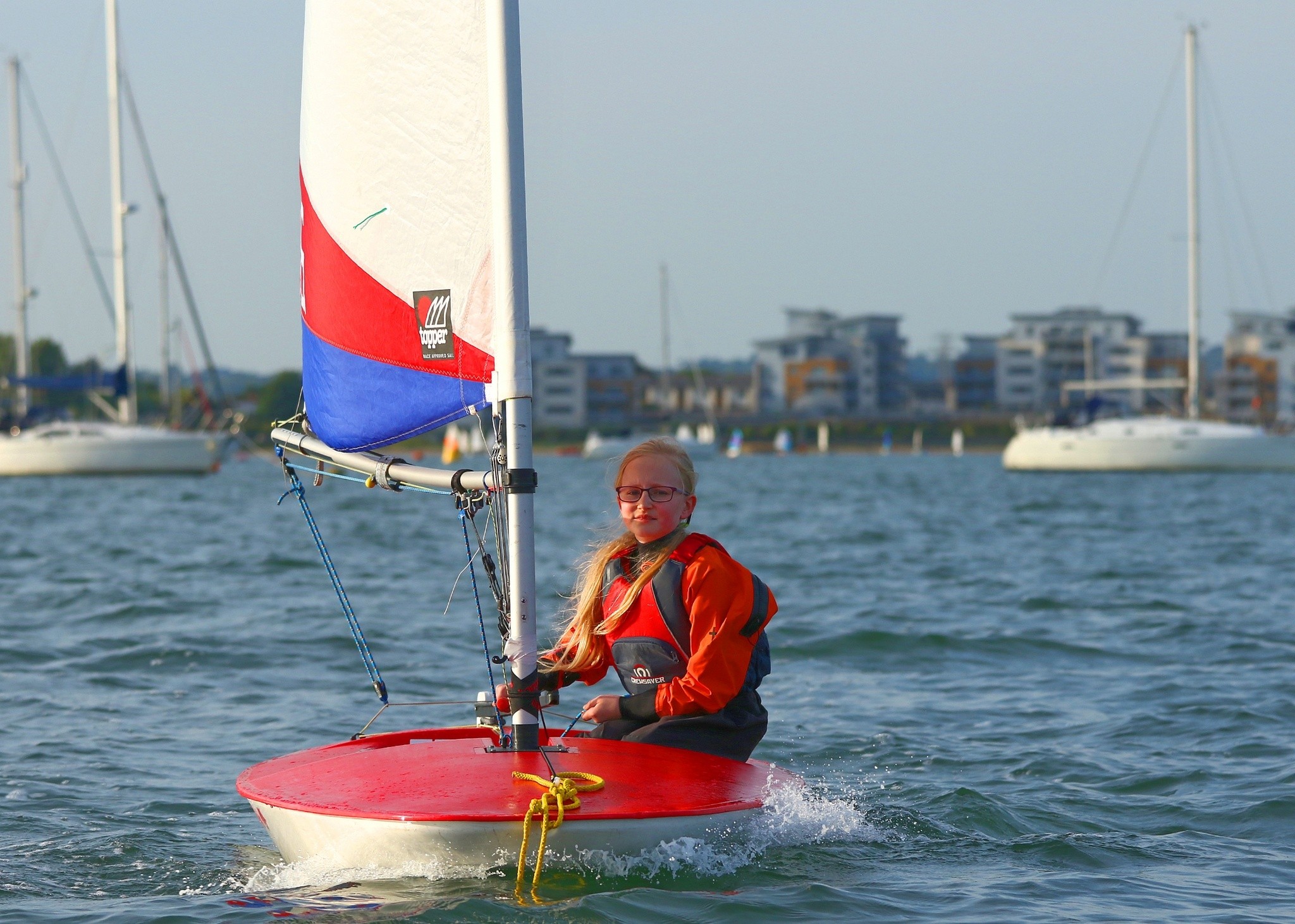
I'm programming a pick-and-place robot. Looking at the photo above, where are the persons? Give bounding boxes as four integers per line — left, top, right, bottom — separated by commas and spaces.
487, 435, 780, 767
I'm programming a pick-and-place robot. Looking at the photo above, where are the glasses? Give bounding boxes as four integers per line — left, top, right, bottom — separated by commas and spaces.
616, 486, 689, 502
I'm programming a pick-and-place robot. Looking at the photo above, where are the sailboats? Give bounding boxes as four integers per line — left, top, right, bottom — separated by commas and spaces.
235, 0, 822, 878
993, 24, 1295, 475
0, 3, 232, 475
